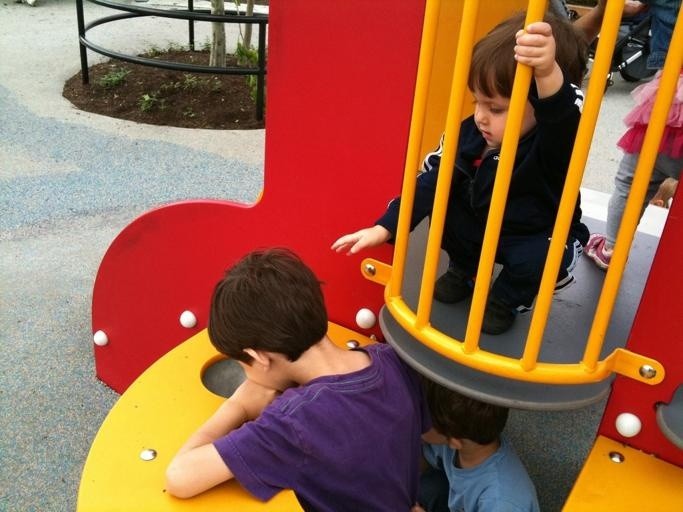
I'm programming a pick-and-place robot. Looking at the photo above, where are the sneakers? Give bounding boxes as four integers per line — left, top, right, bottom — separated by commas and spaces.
480, 288, 517, 337
584, 230, 617, 271
433, 271, 476, 304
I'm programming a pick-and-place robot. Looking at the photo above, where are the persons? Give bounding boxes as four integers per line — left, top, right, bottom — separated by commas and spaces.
330, 7, 588, 334
583, 1, 682, 271
550, 0, 606, 62
650, 178, 678, 209
164, 244, 425, 512
414, 372, 542, 512
